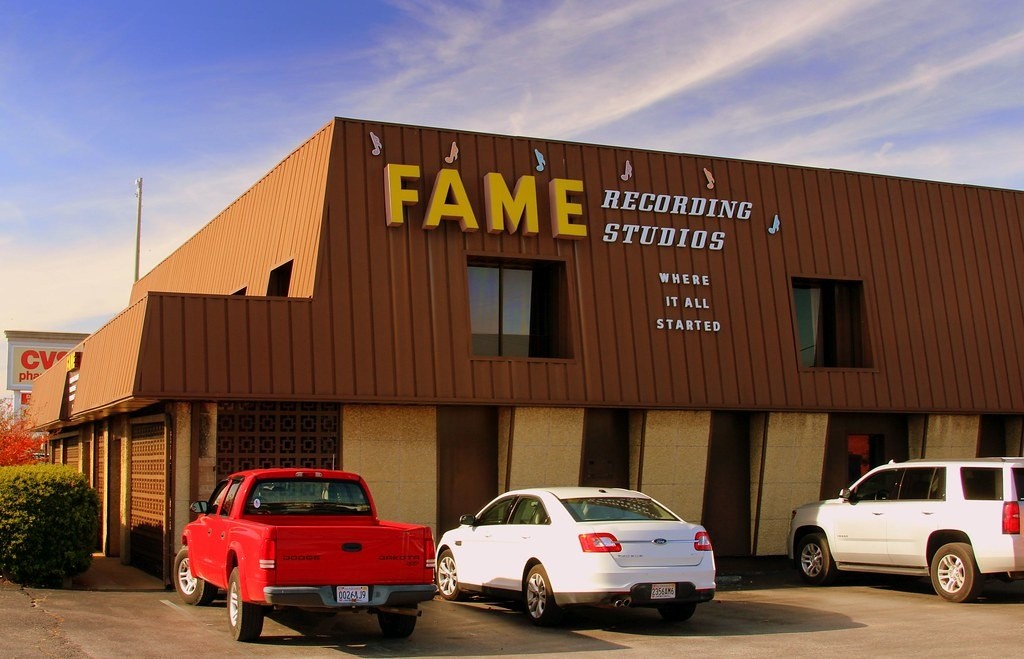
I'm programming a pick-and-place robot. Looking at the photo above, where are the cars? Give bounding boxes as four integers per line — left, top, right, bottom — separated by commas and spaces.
432, 484, 715, 625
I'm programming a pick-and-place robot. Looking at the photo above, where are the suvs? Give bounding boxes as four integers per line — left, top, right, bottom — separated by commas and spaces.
786, 455, 1024, 603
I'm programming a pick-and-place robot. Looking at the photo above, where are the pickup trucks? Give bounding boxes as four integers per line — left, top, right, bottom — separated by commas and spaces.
172, 466, 441, 645
